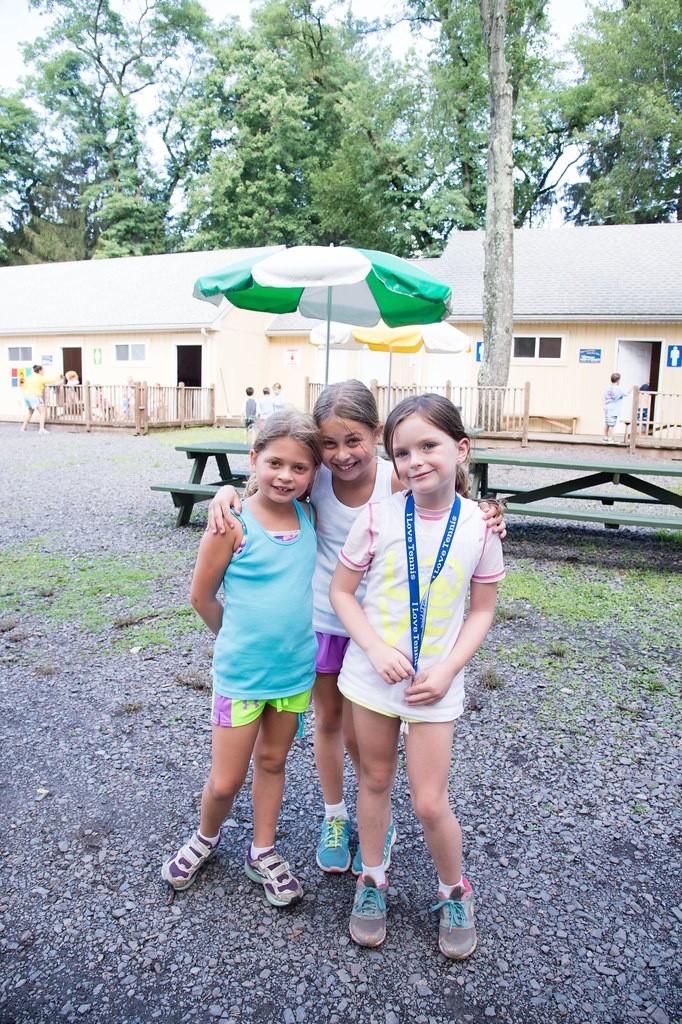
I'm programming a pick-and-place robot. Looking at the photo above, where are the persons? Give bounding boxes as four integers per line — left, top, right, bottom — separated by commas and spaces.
123, 376, 163, 423
329, 394, 506, 960
243, 383, 288, 445
65, 371, 79, 408
20, 365, 64, 435
94, 384, 112, 421
160, 412, 323, 907
210, 379, 507, 876
602, 373, 651, 445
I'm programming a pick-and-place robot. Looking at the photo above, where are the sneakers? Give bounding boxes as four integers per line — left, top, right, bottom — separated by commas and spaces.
244, 841, 304, 908
161, 832, 222, 891
316, 814, 352, 873
429, 878, 477, 960
350, 815, 396, 877
349, 873, 389, 948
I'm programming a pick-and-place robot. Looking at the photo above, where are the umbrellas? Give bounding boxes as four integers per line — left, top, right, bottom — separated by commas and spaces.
193, 243, 452, 390
309, 321, 472, 414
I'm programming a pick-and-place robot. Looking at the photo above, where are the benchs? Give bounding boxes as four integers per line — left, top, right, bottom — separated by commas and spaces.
479, 484, 670, 504
622, 421, 666, 442
503, 502, 682, 528
217, 469, 253, 482
151, 482, 245, 496
503, 415, 578, 434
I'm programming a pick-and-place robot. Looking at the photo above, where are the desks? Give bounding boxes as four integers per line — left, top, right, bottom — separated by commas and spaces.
174, 443, 255, 529
470, 455, 682, 510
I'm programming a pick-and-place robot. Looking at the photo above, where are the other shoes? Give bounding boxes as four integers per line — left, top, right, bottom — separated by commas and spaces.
38, 428, 50, 435
602, 437, 620, 444
19, 427, 33, 433
